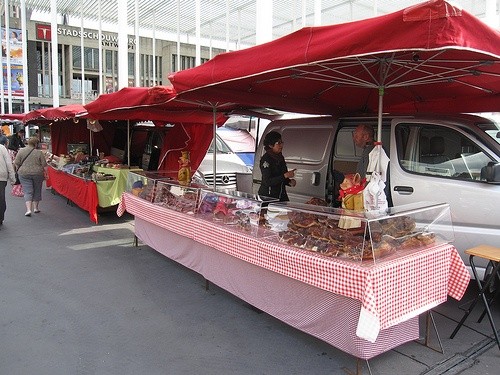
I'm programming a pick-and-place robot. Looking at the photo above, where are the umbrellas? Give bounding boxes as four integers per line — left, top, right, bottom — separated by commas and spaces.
0, 114, 25, 123
82, 87, 230, 192
19, 103, 104, 157
167, 0, 500, 172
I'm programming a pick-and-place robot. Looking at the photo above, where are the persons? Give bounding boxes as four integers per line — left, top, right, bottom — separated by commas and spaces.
0, 135, 16, 225
255, 131, 296, 205
352, 124, 375, 183
15, 136, 49, 215
0, 128, 25, 152
10, 32, 22, 41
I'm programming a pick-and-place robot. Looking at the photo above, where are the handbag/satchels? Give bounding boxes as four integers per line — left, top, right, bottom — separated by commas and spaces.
13, 173, 21, 185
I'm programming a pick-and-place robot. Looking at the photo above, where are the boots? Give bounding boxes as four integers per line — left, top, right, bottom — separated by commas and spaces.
33, 201, 40, 213
24, 201, 31, 216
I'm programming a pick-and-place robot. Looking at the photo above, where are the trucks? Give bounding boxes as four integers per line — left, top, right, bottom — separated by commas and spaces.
110, 124, 258, 193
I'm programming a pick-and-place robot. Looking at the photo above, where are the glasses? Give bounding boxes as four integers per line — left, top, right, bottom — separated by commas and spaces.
275, 141, 284, 146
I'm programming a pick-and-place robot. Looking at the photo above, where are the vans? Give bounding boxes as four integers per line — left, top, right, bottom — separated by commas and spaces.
252, 112, 500, 300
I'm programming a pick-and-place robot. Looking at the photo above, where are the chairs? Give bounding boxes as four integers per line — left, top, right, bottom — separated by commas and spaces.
449, 244, 500, 352
424, 135, 451, 164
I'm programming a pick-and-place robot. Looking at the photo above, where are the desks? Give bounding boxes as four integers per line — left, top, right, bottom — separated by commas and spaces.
44, 162, 143, 224
116, 191, 471, 375
